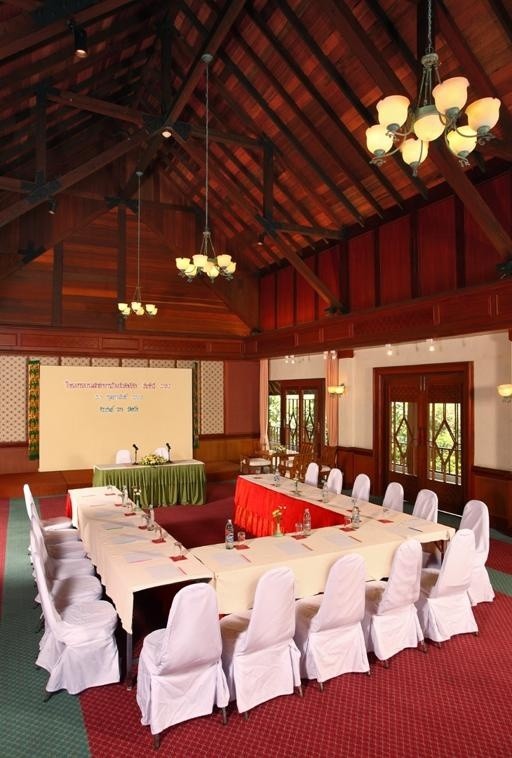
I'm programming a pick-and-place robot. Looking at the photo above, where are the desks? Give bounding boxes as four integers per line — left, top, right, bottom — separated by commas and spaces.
233, 474, 457, 567
92, 459, 208, 508
66, 481, 223, 666
186, 520, 427, 611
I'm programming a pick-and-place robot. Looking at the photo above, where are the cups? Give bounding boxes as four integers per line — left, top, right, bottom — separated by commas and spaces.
155, 527, 162, 542
238, 532, 245, 548
107, 486, 113, 495
295, 522, 303, 537
125, 503, 133, 514
174, 542, 182, 559
344, 516, 351, 528
256, 470, 260, 477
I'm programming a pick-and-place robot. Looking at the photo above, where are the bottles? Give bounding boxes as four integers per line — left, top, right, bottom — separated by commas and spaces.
303, 509, 311, 535
122, 486, 128, 506
147, 505, 154, 531
323, 483, 328, 503
275, 469, 280, 487
352, 503, 360, 529
225, 519, 233, 549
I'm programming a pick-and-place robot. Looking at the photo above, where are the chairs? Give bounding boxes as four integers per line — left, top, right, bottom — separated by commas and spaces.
298, 447, 338, 483
116, 449, 132, 468
153, 449, 170, 465
381, 481, 405, 512
276, 442, 312, 476
23, 512, 87, 589
411, 489, 438, 524
303, 462, 319, 487
458, 500, 496, 607
294, 551, 371, 691
322, 467, 343, 495
238, 444, 271, 476
220, 567, 306, 719
36, 566, 121, 702
135, 582, 230, 748
24, 502, 81, 566
351, 473, 371, 503
419, 529, 480, 649
23, 483, 72, 566
365, 539, 427, 667
29, 552, 102, 658
25, 530, 97, 620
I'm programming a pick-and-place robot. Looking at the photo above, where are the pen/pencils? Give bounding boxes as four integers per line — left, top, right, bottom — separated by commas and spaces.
301, 543, 316, 553
348, 535, 362, 544
83, 495, 97, 497
129, 559, 153, 563
91, 504, 106, 506
177, 566, 189, 577
241, 554, 252, 563
408, 527, 423, 532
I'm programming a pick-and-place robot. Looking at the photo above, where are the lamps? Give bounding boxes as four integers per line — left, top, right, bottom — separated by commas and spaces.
364, 0, 501, 177
117, 172, 158, 319
47, 200, 57, 216
327, 385, 346, 398
162, 128, 172, 139
72, 24, 89, 57
175, 54, 237, 281
258, 232, 265, 246
496, 383, 512, 403
